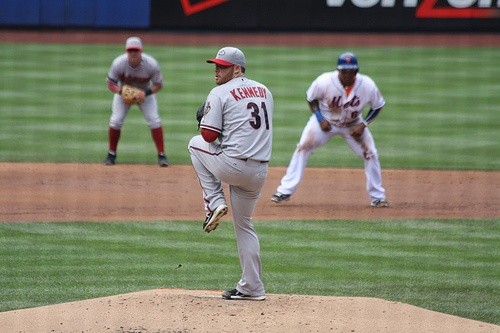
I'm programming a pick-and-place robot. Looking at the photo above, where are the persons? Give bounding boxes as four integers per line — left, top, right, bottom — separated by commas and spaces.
270, 53, 389, 207
105, 38, 169, 166
187, 46, 274, 300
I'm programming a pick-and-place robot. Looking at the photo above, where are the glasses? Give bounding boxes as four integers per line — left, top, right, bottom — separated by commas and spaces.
342, 69, 355, 74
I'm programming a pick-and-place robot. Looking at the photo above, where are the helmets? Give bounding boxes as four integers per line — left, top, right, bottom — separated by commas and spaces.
337, 53, 359, 70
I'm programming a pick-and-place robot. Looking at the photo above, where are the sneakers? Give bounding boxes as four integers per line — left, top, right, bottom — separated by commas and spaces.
105, 152, 116, 165
159, 153, 168, 167
202, 204, 228, 233
222, 289, 267, 301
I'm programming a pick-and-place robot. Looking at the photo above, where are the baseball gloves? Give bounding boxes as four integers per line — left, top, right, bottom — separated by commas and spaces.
120, 85, 145, 104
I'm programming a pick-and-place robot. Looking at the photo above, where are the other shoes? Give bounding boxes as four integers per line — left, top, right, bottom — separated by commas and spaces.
271, 191, 291, 203
371, 199, 388, 207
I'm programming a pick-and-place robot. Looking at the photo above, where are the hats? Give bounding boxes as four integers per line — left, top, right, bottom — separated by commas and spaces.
125, 37, 142, 51
206, 47, 246, 70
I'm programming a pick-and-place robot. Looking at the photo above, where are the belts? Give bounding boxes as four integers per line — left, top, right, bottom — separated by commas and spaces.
240, 158, 269, 163
